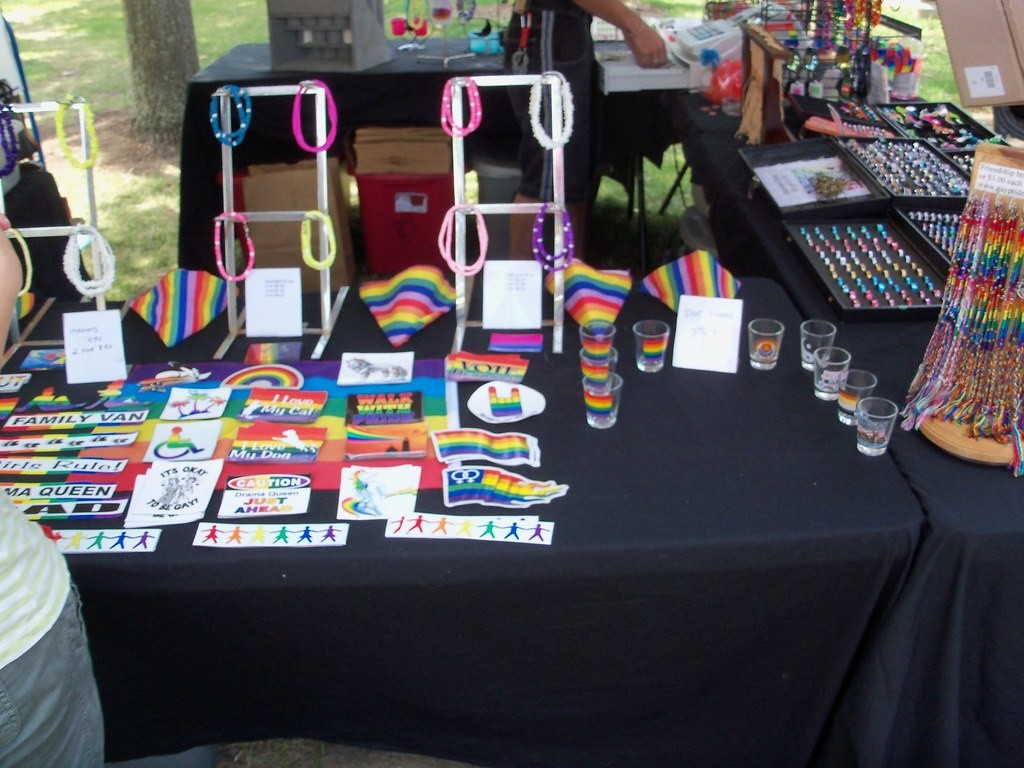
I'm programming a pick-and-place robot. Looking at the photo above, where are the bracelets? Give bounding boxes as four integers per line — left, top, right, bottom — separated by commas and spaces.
0, 104, 18, 176
533, 203, 574, 271
7, 228, 33, 297
57, 95, 98, 169
210, 85, 252, 146
438, 205, 489, 276
215, 212, 255, 281
293, 80, 337, 151
441, 77, 482, 138
301, 211, 336, 270
529, 72, 575, 149
63, 226, 116, 297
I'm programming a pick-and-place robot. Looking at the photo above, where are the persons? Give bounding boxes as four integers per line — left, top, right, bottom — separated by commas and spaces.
0, 213, 103, 768
504, 0, 666, 320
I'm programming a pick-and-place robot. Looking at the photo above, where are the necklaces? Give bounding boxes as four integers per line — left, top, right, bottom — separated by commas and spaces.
800, 0, 884, 48
900, 196, 1024, 477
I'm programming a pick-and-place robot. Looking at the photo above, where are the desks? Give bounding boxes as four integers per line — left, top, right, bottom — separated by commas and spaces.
663, 94, 1024, 768
0, 276, 928, 768
178, 35, 690, 272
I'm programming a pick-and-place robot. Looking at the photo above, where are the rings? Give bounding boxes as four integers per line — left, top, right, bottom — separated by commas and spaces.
799, 224, 941, 307
843, 103, 973, 257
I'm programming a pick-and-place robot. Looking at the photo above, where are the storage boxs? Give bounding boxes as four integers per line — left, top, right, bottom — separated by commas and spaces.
252, 227, 355, 293
345, 164, 471, 276
244, 158, 354, 249
934, 0, 1024, 108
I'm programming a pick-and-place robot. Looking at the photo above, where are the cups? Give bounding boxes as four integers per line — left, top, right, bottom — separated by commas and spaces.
748, 318, 784, 371
838, 369, 877, 426
579, 319, 623, 429
813, 346, 851, 401
632, 319, 670, 373
856, 396, 898, 456
801, 319, 836, 372
